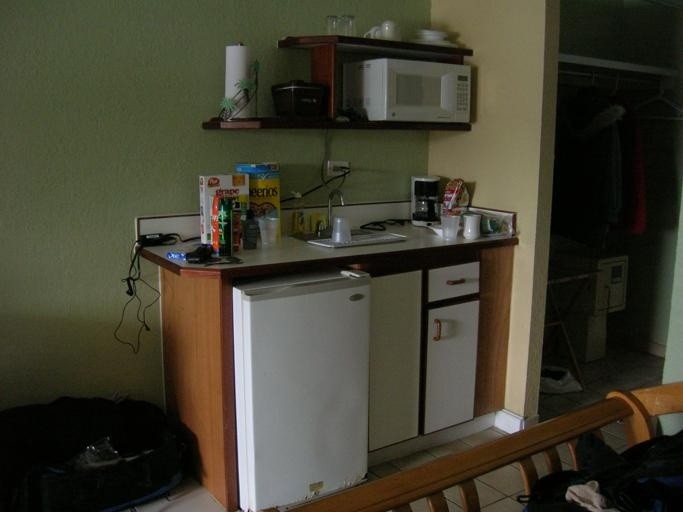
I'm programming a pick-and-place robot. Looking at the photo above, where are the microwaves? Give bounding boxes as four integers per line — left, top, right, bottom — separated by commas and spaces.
342, 58, 471, 123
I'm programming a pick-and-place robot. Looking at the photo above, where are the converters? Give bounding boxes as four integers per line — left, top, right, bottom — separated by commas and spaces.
137, 233, 163, 245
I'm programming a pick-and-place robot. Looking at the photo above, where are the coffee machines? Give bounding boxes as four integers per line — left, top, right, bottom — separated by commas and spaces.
410, 174, 441, 227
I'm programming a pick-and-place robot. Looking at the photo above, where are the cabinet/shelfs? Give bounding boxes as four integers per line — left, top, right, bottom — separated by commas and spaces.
367, 260, 422, 466
422, 250, 480, 455
202, 35, 471, 130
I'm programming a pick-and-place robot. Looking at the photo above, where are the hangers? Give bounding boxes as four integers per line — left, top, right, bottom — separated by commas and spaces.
635, 77, 682, 121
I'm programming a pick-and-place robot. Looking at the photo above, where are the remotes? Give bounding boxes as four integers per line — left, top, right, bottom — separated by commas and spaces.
188, 244, 212, 263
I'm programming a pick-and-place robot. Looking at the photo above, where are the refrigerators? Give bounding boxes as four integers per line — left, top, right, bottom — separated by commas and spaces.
229, 270, 373, 512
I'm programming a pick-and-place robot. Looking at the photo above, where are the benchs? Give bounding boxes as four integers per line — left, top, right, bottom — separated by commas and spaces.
291, 383, 683, 511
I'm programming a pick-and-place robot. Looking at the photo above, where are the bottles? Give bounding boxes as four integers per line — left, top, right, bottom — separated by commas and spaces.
211, 190, 257, 258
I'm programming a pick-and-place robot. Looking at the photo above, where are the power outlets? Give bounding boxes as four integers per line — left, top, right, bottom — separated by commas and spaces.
327, 161, 350, 177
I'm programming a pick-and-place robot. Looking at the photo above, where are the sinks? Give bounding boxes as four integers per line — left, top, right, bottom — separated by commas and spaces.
289, 228, 373, 242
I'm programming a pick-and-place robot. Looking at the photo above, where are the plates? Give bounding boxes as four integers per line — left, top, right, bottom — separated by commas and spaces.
408, 29, 458, 48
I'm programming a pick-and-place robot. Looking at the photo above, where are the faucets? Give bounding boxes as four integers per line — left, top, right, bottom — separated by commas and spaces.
328, 190, 344, 232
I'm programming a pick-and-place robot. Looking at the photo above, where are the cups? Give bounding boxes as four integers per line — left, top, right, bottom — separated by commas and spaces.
463, 214, 482, 240
331, 217, 351, 243
326, 15, 356, 37
363, 19, 401, 41
258, 216, 281, 249
440, 215, 460, 241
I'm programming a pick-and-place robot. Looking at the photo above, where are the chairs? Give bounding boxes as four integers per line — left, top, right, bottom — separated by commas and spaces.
544, 249, 606, 394
